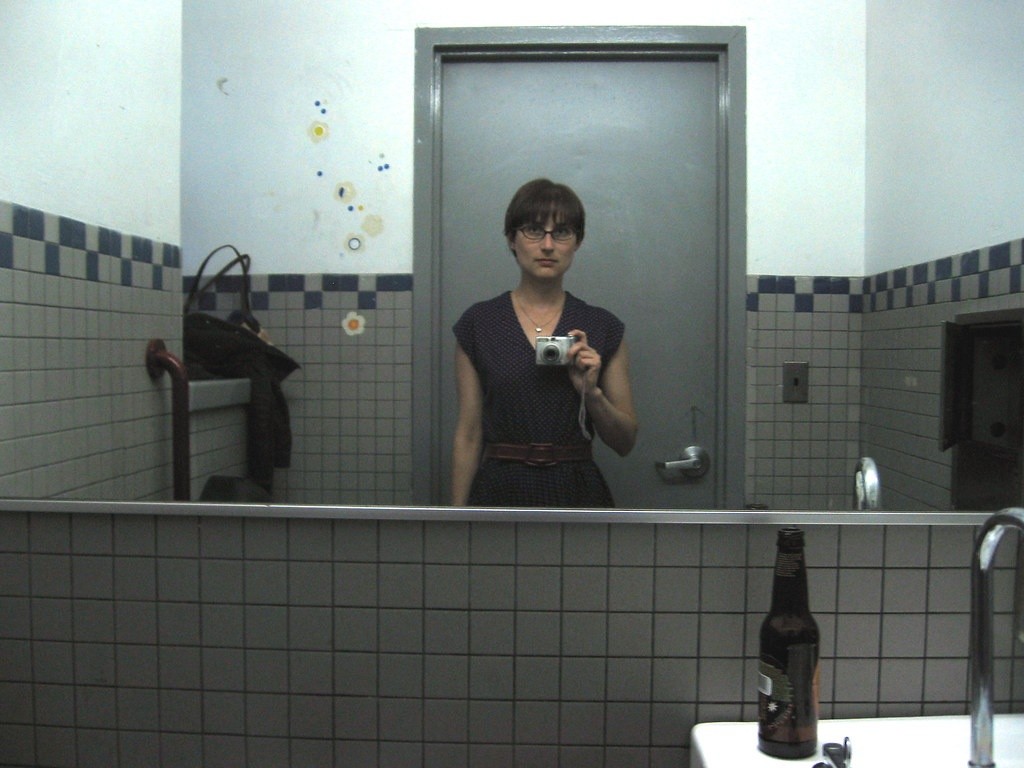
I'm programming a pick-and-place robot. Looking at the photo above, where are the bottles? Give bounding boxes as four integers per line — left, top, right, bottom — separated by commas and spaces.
747, 503, 768, 510
759, 527, 820, 760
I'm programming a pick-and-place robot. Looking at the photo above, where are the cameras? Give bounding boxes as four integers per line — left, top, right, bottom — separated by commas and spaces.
534, 335, 578, 367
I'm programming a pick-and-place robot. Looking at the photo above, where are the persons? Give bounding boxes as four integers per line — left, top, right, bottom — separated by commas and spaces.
450, 178, 637, 508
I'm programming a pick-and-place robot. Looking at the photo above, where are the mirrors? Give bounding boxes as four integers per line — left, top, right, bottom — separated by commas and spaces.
0, 0, 1024, 524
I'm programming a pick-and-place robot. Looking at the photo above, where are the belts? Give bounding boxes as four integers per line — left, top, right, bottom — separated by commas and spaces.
482, 441, 593, 467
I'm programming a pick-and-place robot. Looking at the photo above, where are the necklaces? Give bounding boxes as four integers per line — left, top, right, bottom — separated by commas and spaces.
514, 289, 567, 333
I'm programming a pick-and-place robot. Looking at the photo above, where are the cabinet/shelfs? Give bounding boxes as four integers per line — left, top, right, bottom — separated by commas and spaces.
938, 308, 1024, 513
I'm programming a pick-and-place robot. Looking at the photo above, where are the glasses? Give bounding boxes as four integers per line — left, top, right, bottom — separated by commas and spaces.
515, 225, 577, 241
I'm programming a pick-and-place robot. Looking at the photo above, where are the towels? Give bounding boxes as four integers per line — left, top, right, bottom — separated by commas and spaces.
246, 335, 292, 502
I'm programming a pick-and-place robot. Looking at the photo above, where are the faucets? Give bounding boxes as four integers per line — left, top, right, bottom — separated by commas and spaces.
851, 457, 882, 512
966, 507, 1024, 768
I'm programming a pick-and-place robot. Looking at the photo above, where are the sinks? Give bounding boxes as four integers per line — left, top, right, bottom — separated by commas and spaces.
684, 711, 1024, 768
